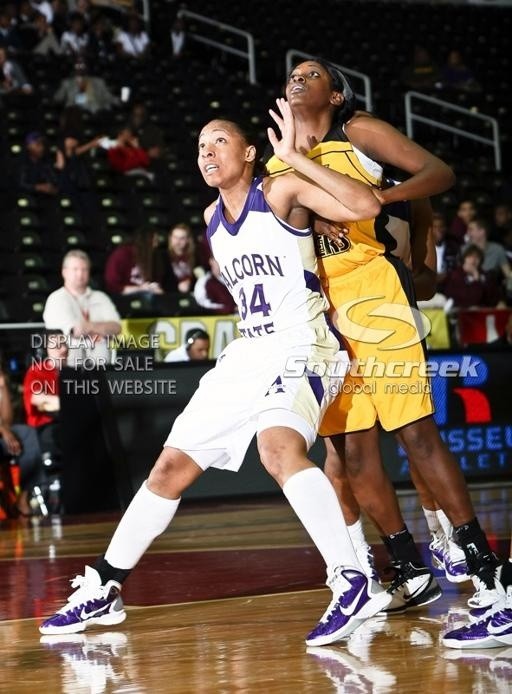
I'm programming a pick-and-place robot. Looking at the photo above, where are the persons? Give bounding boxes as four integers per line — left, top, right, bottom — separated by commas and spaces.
468, 535, 512, 606
0, 220, 241, 526
262, 56, 505, 614
443, 581, 512, 651
0, 0, 192, 199
310, 100, 477, 585
394, 42, 512, 353
36, 97, 393, 651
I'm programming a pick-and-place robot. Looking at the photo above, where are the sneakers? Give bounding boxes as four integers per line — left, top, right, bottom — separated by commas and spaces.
427, 530, 470, 585
373, 558, 444, 617
37, 564, 128, 640
304, 567, 395, 648
442, 550, 512, 650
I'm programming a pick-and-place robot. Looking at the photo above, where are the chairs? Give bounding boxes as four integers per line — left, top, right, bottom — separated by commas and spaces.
1, 0, 511, 338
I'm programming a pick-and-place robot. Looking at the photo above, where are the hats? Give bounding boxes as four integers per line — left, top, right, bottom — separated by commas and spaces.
27, 131, 45, 143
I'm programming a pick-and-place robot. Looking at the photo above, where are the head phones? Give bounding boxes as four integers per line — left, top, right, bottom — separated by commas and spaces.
33, 329, 51, 361
186, 330, 204, 350
